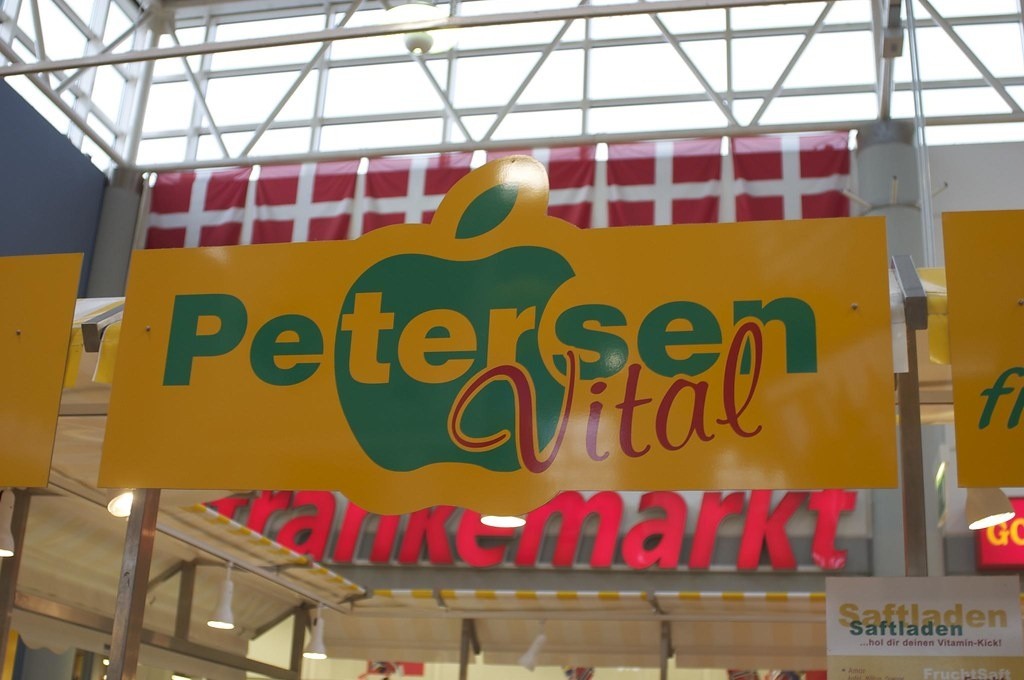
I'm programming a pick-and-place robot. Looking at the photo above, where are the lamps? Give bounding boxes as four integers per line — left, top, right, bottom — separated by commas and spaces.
965, 487, 1014, 531
301, 604, 327, 660
206, 562, 235, 628
516, 633, 547, 670
105, 489, 133, 517
